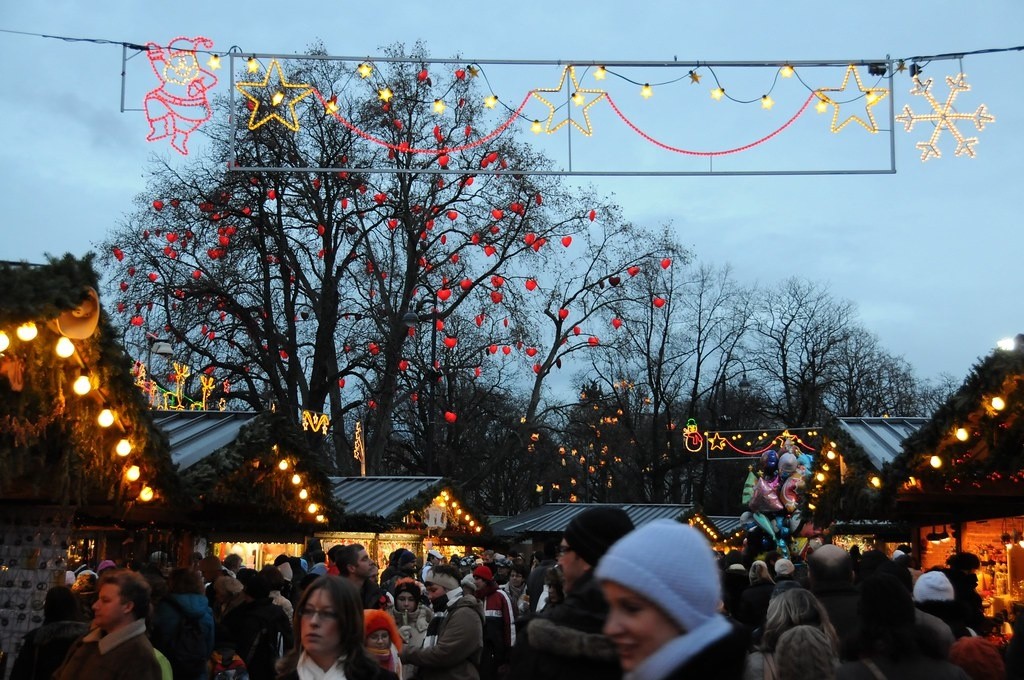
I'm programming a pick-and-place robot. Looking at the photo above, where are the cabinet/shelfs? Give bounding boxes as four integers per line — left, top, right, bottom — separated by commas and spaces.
0, 500, 128, 680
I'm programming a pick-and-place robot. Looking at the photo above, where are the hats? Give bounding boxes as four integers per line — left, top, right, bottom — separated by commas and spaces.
365, 617, 392, 638
892, 550, 904, 560
395, 576, 421, 605
592, 517, 722, 633
913, 571, 955, 602
59, 570, 76, 587
564, 505, 635, 569
429, 549, 444, 558
98, 560, 116, 573
77, 570, 98, 579
460, 573, 476, 591
749, 561, 767, 581
949, 636, 1006, 680
398, 550, 415, 566
774, 557, 795, 577
473, 566, 492, 581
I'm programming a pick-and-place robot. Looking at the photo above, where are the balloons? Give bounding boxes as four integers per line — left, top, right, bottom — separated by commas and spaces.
741, 439, 813, 540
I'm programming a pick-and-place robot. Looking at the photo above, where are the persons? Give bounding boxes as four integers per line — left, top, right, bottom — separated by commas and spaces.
743, 588, 839, 680
398, 564, 485, 680
362, 609, 403, 680
915, 552, 985, 639
8, 585, 77, 680
806, 544, 895, 663
151, 567, 215, 680
473, 566, 517, 680
216, 573, 294, 680
206, 641, 249, 680
773, 626, 837, 680
948, 635, 1006, 680
52, 568, 173, 680
878, 561, 955, 660
70, 544, 922, 680
1004, 603, 1024, 680
835, 573, 972, 680
595, 518, 751, 680
277, 574, 400, 680
506, 507, 635, 680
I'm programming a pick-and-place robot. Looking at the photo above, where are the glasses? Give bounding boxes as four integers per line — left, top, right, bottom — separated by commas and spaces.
367, 632, 390, 643
510, 574, 522, 578
554, 545, 571, 559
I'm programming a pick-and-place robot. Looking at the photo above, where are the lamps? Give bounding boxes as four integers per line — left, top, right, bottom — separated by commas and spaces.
1000, 518, 1024, 549
926, 524, 951, 545
